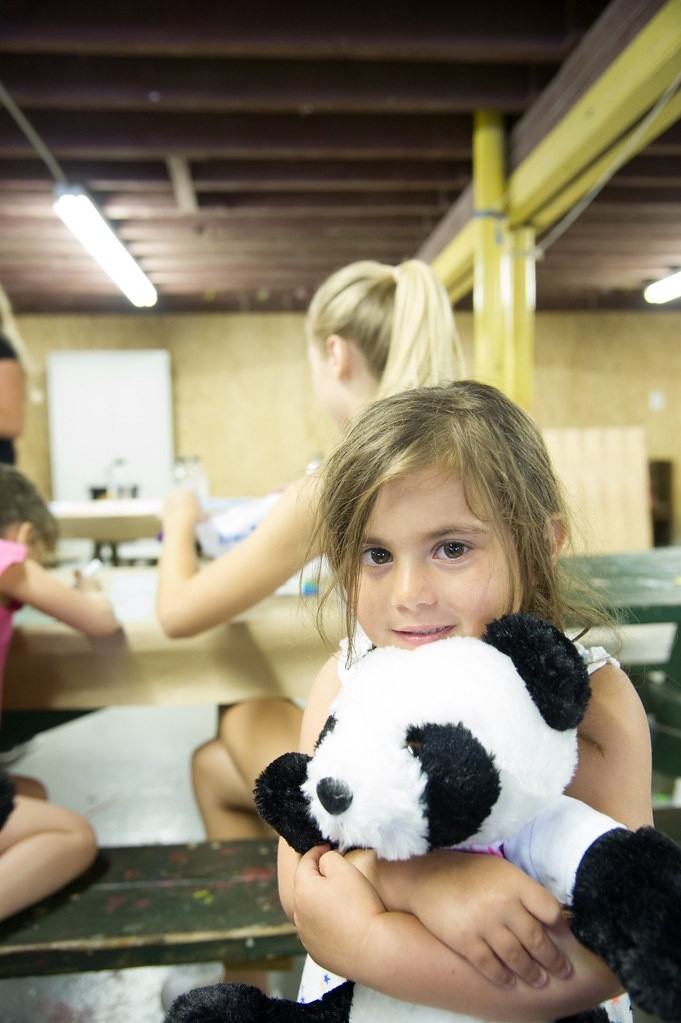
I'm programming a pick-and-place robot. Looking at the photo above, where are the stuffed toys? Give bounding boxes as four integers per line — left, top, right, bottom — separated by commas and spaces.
166, 612, 680, 1023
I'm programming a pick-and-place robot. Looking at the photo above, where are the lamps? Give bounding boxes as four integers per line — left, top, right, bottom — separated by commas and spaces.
52, 180, 160, 308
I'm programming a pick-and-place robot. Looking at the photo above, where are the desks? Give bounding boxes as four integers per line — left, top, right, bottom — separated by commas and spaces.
45, 516, 161, 540
560, 551, 681, 778
3, 580, 354, 710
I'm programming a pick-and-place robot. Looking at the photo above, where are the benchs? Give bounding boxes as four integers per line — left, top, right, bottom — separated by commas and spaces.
0, 835, 304, 981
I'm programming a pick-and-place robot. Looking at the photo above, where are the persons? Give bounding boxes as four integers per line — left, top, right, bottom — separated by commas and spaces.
157, 260, 473, 998
0, 330, 120, 922
276, 378, 657, 1023
